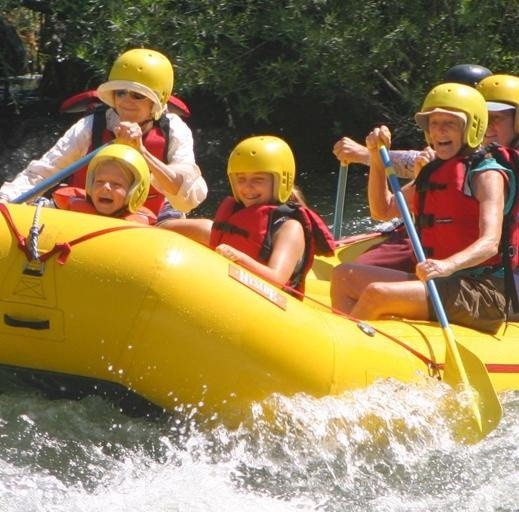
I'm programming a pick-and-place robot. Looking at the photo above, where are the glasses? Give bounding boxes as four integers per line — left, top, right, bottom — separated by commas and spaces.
113, 89, 148, 100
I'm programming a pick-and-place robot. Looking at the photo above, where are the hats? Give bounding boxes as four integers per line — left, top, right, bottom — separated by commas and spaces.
97, 80, 161, 123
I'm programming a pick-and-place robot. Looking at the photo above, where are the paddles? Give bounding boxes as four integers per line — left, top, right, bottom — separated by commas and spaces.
366, 128, 502, 447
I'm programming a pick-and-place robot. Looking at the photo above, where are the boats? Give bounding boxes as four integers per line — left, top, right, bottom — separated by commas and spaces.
0, 201, 519, 439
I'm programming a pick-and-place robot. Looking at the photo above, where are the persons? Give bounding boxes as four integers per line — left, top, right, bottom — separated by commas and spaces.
0, 49, 213, 247
333, 73, 518, 181
33, 144, 158, 223
330, 82, 519, 334
209, 135, 335, 302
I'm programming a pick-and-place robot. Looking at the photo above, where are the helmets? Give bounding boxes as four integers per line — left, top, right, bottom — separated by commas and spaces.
421, 63, 518, 147
86, 144, 150, 214
97, 48, 174, 122
227, 135, 296, 203
